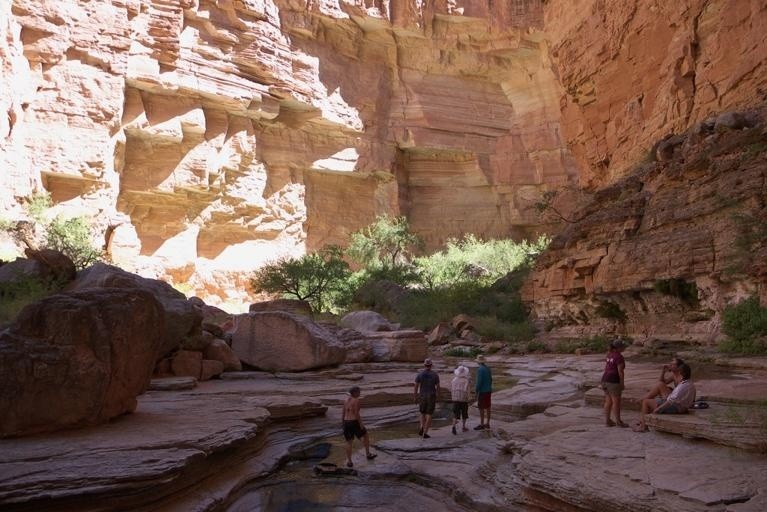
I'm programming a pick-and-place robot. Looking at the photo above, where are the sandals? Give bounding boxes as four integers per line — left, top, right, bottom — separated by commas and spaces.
367, 452, 377, 458
347, 461, 353, 467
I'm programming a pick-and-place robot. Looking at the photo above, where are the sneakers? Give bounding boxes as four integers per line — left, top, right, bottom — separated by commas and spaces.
419, 426, 423, 435
473, 425, 484, 430
616, 421, 629, 428
452, 426, 456, 434
606, 419, 616, 426
462, 428, 468, 432
484, 424, 489, 428
424, 434, 430, 438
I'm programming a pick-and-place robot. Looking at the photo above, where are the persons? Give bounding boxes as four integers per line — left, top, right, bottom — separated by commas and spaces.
339, 385, 378, 469
412, 357, 440, 439
450, 363, 472, 436
473, 353, 493, 432
599, 338, 629, 429
629, 357, 684, 407
630, 363, 696, 433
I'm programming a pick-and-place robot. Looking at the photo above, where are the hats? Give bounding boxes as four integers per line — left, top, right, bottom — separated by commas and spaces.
473, 355, 487, 363
348, 386, 359, 394
453, 365, 469, 377
613, 339, 625, 348
424, 359, 433, 366
